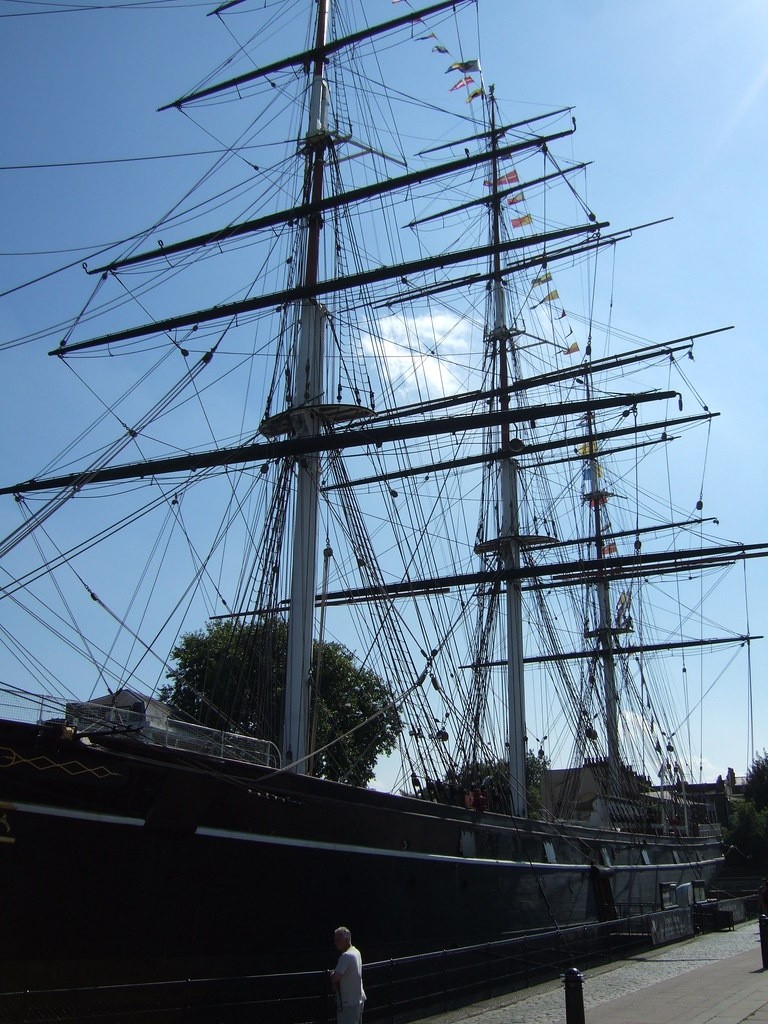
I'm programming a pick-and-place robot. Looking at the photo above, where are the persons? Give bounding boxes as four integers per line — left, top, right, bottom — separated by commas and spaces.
330, 927, 367, 1024
759, 878, 768, 913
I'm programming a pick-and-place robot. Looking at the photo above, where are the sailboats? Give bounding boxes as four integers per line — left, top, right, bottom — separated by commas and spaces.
0, 0, 768, 1024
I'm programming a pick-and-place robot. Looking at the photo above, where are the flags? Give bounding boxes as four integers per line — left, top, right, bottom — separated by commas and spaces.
577, 421, 617, 554
541, 290, 559, 301
531, 272, 552, 288
496, 170, 519, 184
555, 309, 579, 355
412, 17, 484, 103
616, 593, 633, 628
511, 214, 533, 229
507, 192, 524, 204
